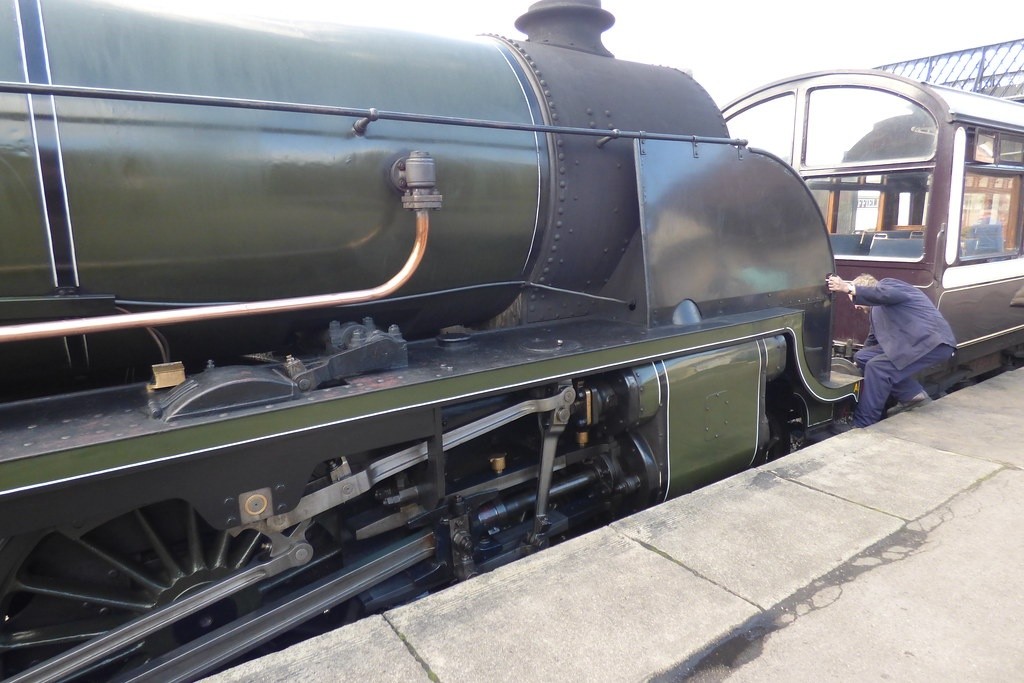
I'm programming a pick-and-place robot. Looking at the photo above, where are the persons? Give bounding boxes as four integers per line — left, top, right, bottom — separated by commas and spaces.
827, 272, 958, 435
964, 198, 1004, 257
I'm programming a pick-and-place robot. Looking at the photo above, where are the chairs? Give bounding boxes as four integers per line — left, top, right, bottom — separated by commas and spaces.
829, 225, 1003, 258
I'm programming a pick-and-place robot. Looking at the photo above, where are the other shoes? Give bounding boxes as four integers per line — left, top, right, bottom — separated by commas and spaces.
831, 416, 865, 434
887, 389, 931, 417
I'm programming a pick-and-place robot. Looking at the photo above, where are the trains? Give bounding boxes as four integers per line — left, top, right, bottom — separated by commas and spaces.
0, 0, 1024, 682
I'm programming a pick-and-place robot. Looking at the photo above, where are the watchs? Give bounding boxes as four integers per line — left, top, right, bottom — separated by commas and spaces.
847, 283, 853, 294
